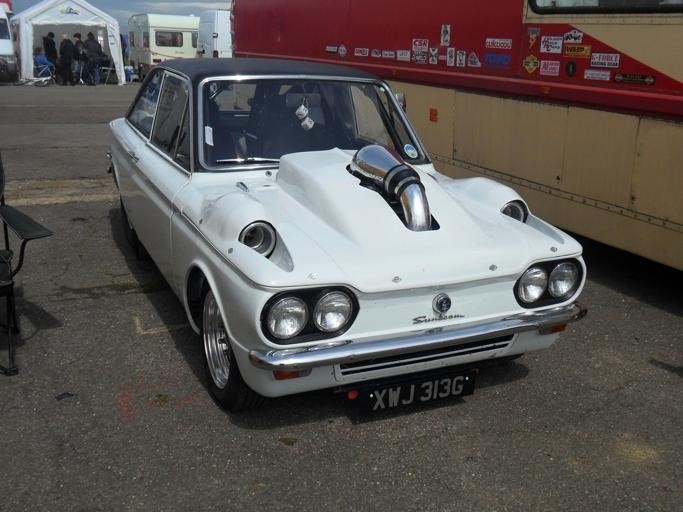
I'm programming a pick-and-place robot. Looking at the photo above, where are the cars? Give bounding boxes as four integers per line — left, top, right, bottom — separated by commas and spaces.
107, 54, 588, 423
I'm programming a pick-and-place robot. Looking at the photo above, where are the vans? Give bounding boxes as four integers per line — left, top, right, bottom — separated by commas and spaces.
0, 3, 18, 84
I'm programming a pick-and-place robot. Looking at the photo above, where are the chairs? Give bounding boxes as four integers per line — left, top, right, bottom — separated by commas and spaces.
32, 56, 54, 77
205, 99, 236, 168
0, 152, 54, 375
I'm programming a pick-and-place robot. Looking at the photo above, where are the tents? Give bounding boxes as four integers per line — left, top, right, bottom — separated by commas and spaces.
8, 0, 127, 86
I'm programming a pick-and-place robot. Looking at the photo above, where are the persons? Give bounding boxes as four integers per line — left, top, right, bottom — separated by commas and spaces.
35, 32, 109, 86
